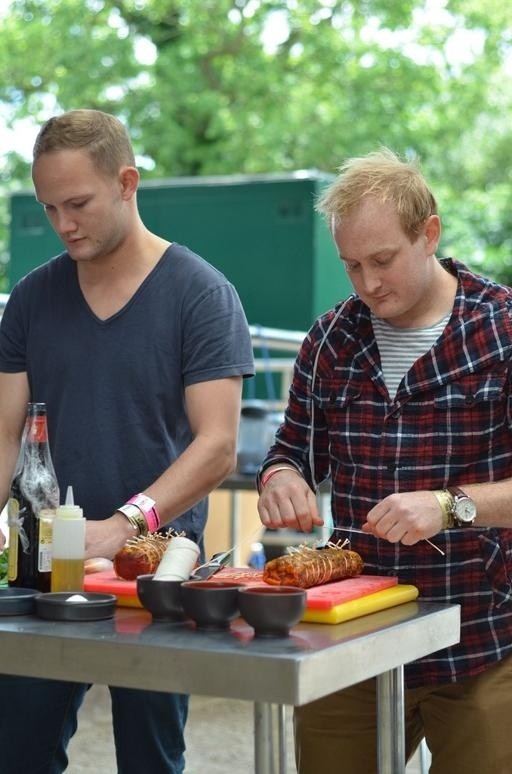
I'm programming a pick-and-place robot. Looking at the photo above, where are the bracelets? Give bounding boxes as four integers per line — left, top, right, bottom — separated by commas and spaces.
114, 503, 148, 538
431, 488, 456, 529
257, 462, 302, 495
126, 493, 160, 535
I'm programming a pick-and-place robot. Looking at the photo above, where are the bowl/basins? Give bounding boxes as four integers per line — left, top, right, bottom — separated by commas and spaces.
236, 585, 307, 639
180, 579, 249, 631
135, 574, 201, 623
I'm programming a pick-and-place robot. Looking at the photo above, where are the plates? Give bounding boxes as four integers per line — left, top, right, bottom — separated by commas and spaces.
0, 587, 41, 616
36, 592, 116, 620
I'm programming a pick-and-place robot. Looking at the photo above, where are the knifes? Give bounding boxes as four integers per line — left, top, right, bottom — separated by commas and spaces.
193, 550, 232, 581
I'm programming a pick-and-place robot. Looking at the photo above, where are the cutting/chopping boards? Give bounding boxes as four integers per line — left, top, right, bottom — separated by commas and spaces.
82, 559, 419, 624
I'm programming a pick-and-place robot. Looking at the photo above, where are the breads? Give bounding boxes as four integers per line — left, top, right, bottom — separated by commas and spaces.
113, 538, 201, 580
261, 550, 363, 587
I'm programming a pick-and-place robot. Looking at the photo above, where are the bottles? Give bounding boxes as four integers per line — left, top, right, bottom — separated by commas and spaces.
6, 401, 86, 591
152, 537, 201, 581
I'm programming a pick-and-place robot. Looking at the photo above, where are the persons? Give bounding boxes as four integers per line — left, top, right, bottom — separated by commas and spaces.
254, 147, 511, 773
0, 109, 256, 773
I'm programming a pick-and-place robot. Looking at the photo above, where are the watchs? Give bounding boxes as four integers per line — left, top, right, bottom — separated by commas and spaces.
444, 486, 477, 528
